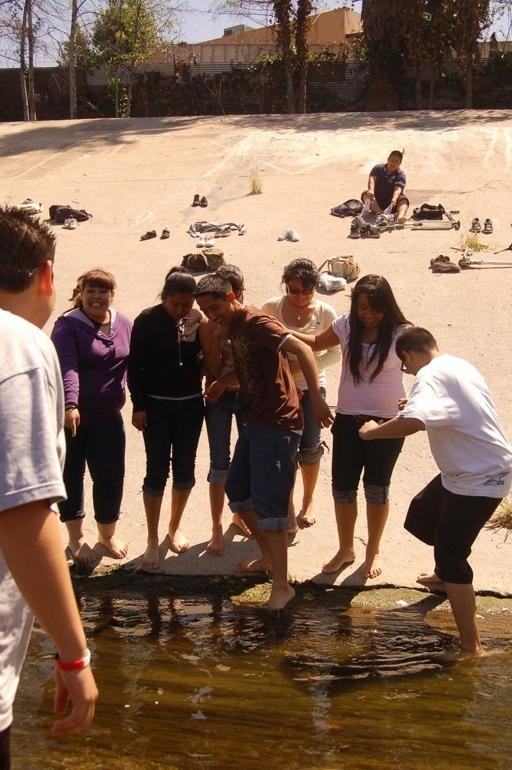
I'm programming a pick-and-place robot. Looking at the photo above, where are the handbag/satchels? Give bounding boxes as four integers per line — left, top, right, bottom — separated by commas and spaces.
49, 205, 71, 220
413, 204, 444, 219
430, 255, 459, 273
55, 209, 88, 222
16, 199, 42, 214
315, 272, 346, 292
332, 199, 364, 217
318, 256, 359, 282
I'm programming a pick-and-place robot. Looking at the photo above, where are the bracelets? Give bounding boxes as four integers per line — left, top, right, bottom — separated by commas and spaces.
57, 650, 96, 670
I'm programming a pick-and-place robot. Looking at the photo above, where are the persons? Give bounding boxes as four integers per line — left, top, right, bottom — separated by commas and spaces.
355, 327, 512, 674
0, 196, 106, 770
313, 276, 416, 577
122, 270, 207, 568
46, 266, 133, 565
186, 266, 259, 557
354, 150, 410, 223
207, 273, 333, 611
255, 260, 347, 532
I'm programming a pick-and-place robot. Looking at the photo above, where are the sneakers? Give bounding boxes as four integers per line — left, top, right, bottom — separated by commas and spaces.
484, 219, 493, 233
350, 221, 361, 238
70, 218, 77, 229
201, 197, 208, 206
214, 229, 223, 237
193, 194, 200, 206
361, 224, 381, 237
197, 237, 205, 247
162, 227, 169, 238
206, 237, 215, 247
63, 219, 69, 228
289, 228, 298, 242
473, 218, 480, 232
222, 227, 230, 237
141, 230, 156, 240
277, 229, 288, 241
